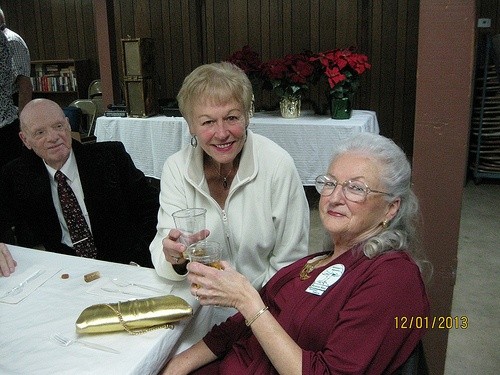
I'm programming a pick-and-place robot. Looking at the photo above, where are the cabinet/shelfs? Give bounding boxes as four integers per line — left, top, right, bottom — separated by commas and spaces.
30, 58, 95, 107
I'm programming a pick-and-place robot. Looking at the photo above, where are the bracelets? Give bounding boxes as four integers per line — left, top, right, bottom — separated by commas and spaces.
246, 306, 269, 326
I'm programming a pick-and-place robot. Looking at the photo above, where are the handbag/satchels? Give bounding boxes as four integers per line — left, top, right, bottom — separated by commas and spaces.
74, 294, 195, 337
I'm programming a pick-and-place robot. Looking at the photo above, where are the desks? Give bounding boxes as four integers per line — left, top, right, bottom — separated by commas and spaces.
94, 110, 381, 187
0, 243, 201, 375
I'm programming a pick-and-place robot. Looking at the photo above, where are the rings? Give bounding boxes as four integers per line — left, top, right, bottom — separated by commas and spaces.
193, 283, 200, 288
171, 255, 183, 262
195, 291, 199, 300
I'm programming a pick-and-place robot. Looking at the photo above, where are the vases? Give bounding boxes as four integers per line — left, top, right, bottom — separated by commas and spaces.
280, 94, 301, 118
329, 85, 351, 119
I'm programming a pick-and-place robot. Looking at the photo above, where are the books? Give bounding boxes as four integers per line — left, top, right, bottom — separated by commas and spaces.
30, 63, 77, 92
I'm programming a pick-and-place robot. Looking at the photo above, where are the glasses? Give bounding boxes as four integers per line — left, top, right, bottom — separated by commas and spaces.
314, 174, 394, 203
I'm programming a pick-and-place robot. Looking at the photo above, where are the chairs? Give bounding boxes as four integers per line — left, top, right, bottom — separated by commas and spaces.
67, 80, 104, 137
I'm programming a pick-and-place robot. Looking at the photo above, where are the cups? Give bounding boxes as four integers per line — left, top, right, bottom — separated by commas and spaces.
185, 241, 224, 308
172, 208, 207, 259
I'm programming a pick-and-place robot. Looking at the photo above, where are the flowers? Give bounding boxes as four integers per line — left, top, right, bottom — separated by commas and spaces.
260, 45, 372, 98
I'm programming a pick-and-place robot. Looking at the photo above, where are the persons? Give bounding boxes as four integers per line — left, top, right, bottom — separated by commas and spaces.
0, 10, 29, 235
158, 132, 432, 375
0, 98, 155, 278
148, 62, 310, 291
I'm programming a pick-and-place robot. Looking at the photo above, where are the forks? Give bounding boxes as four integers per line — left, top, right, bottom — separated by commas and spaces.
54, 333, 119, 354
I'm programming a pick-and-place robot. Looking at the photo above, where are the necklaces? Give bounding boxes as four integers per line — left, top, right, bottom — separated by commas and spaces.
215, 169, 233, 187
298, 254, 331, 280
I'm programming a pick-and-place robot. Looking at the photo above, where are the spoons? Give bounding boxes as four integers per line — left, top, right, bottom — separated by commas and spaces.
113, 278, 166, 294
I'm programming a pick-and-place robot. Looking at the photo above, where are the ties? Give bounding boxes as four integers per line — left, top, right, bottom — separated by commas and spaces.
54, 170, 97, 259
